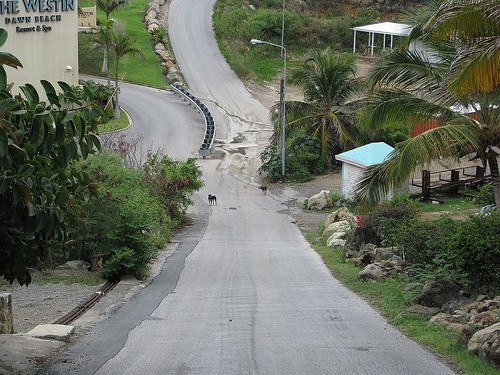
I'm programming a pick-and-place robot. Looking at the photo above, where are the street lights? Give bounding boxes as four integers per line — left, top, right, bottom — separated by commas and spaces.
250, 37, 288, 178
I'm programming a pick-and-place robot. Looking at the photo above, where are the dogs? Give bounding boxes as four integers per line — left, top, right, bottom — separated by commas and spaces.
259, 186, 267, 195
208, 194, 217, 205
